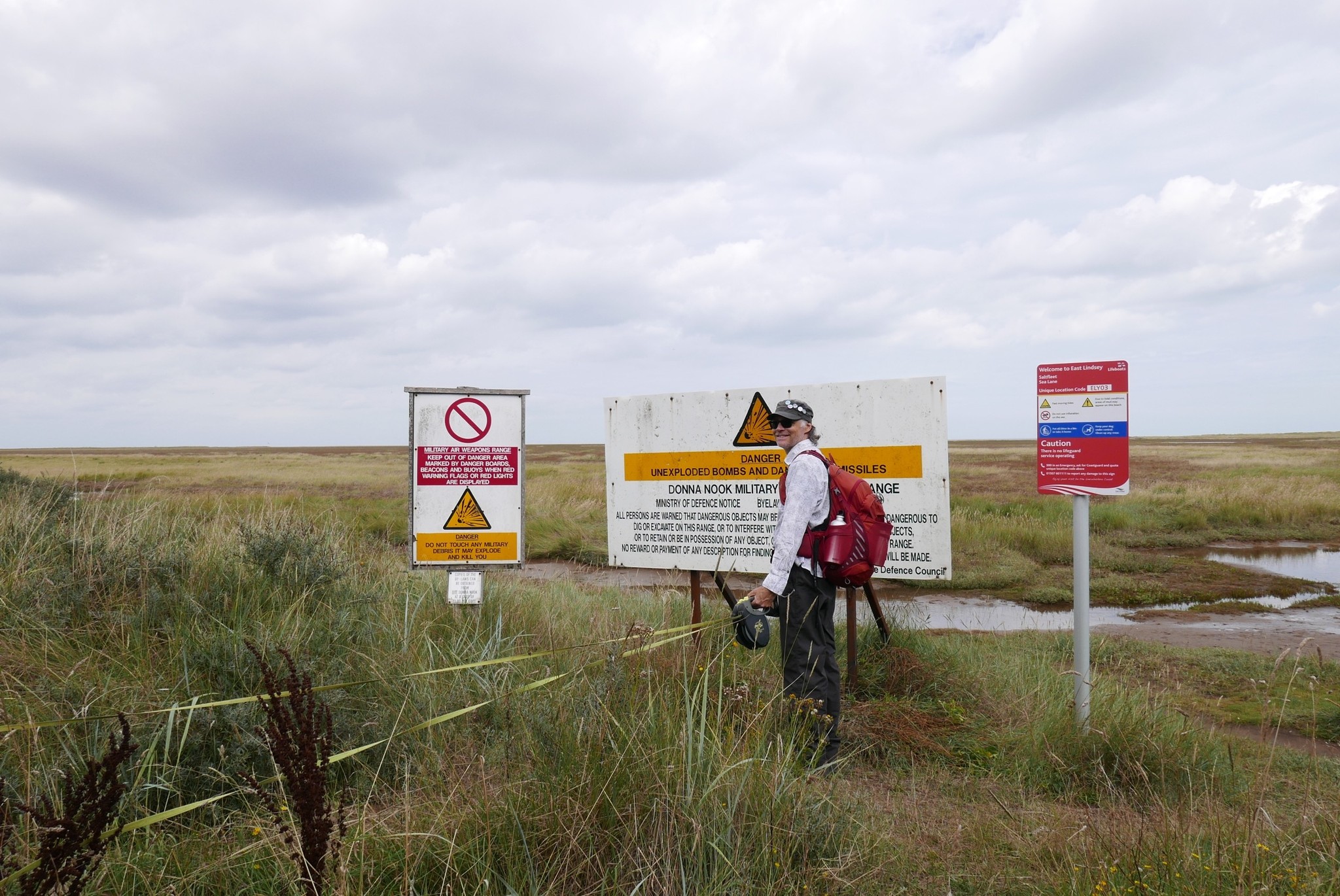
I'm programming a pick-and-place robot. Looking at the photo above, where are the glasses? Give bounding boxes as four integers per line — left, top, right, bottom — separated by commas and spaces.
769, 418, 792, 429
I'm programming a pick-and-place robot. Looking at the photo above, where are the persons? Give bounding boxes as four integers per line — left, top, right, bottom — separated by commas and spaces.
748, 399, 842, 772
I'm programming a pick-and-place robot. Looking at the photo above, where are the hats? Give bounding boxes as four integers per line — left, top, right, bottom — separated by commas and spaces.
767, 399, 814, 424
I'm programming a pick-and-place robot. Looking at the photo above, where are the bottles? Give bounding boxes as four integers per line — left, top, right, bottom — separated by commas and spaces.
825, 510, 846, 565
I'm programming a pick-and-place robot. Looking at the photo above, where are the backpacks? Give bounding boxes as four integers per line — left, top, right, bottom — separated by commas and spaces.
779, 449, 894, 587
